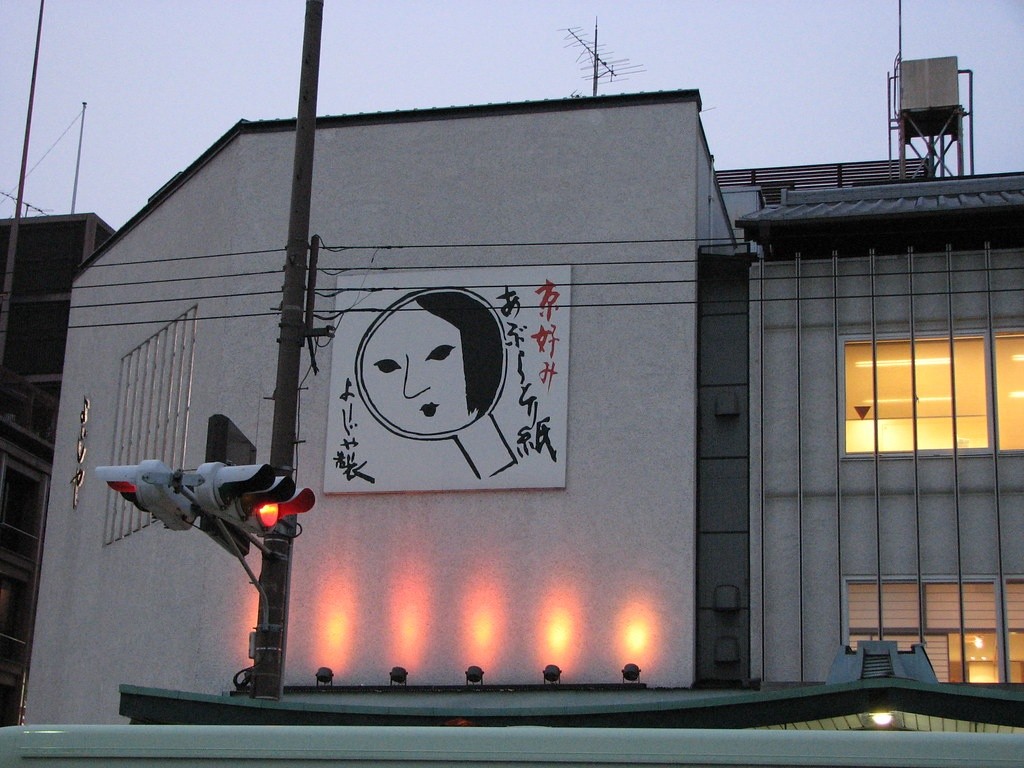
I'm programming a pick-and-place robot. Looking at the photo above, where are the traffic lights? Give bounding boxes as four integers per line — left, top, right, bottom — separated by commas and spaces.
194, 460, 317, 538
94, 459, 197, 531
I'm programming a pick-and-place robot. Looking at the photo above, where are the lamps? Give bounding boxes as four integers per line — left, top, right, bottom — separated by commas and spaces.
389, 667, 408, 687
465, 666, 484, 686
315, 667, 334, 687
542, 665, 562, 685
621, 664, 642, 684
